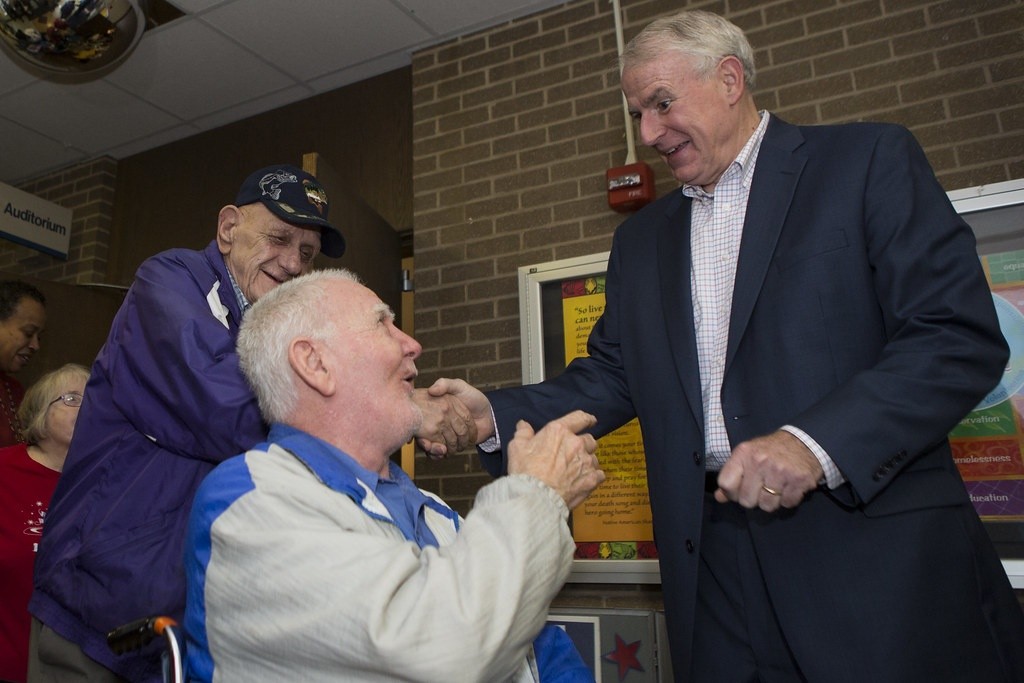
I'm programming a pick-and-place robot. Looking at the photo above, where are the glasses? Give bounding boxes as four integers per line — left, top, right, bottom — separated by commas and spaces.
50, 392, 84, 407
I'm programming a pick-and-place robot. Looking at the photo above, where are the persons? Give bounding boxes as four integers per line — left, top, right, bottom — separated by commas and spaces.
0, 164, 607, 683
417, 11, 1023, 683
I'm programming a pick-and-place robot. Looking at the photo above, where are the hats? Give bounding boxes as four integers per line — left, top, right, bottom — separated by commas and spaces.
233, 164, 345, 259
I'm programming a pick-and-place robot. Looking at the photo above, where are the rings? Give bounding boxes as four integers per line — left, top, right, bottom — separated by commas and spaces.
762, 484, 778, 497
